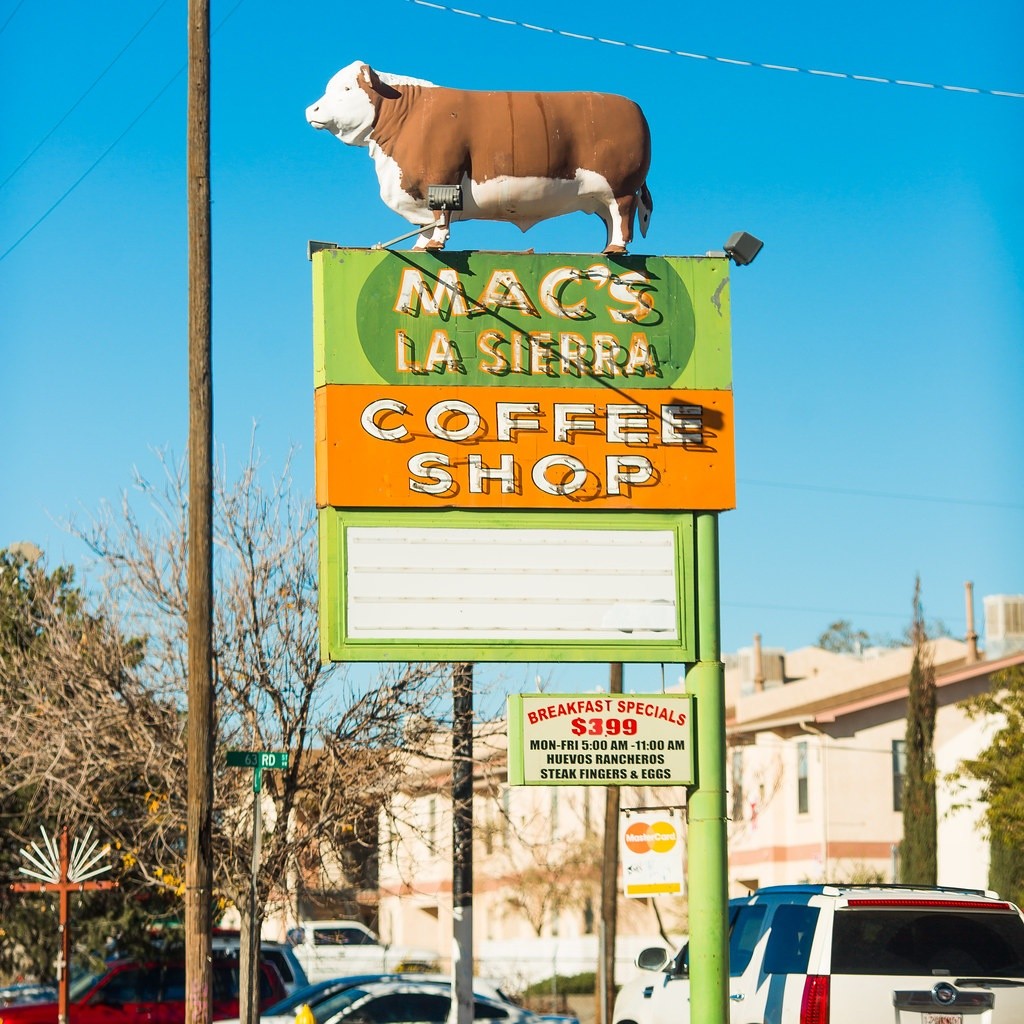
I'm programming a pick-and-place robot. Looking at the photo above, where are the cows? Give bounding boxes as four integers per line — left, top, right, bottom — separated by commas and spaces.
303, 57, 654, 256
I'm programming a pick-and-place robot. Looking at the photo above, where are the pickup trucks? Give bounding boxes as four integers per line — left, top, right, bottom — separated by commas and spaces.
282, 918, 398, 984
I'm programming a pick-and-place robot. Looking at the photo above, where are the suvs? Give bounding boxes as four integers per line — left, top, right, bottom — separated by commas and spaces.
0, 938, 311, 1003
613, 881, 1024, 1024
0, 955, 289, 1024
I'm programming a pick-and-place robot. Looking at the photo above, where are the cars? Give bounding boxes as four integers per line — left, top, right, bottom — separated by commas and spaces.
217, 971, 580, 1024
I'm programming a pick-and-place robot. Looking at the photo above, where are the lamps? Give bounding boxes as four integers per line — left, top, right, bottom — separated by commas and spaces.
429, 183, 462, 226
722, 230, 765, 268
